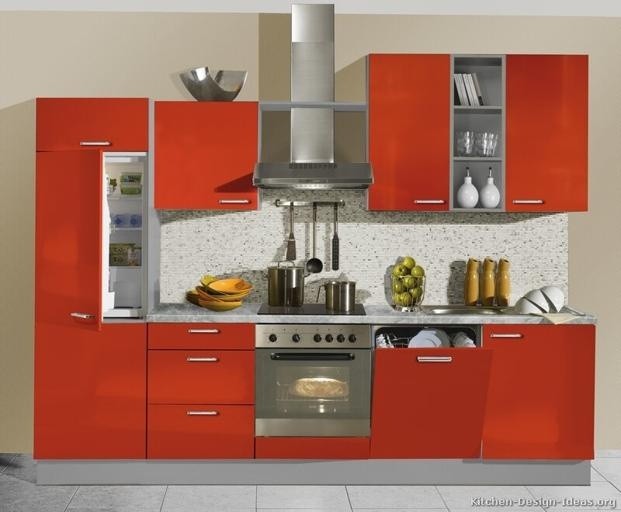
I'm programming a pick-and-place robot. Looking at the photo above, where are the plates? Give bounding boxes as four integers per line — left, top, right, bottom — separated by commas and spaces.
409, 335, 442, 347
186, 276, 253, 311
420, 328, 452, 348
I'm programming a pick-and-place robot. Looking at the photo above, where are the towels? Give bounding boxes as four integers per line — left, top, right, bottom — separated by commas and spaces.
543, 311, 580, 326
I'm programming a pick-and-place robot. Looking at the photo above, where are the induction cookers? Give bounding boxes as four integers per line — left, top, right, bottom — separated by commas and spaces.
254, 304, 371, 324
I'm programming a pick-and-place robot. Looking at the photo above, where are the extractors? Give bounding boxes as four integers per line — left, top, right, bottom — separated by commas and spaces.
253, 103, 374, 190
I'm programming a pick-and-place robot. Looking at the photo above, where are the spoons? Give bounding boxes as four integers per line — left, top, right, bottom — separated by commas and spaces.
306, 203, 323, 273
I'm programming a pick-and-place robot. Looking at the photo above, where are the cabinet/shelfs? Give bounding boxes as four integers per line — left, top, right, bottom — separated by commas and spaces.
483, 324, 597, 462
148, 322, 256, 459
363, 50, 591, 215
34, 95, 151, 154
31, 153, 148, 324
33, 325, 146, 460
370, 326, 483, 462
153, 99, 262, 211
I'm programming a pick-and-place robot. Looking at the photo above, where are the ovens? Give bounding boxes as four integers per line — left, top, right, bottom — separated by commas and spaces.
255, 324, 371, 439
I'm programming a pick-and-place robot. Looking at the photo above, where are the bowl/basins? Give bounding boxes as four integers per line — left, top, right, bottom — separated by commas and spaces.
542, 286, 565, 312
514, 298, 544, 315
524, 290, 551, 313
450, 330, 475, 347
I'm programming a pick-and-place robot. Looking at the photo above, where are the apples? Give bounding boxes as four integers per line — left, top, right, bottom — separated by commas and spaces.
394, 293, 400, 302
401, 256, 415, 270
391, 279, 404, 293
403, 274, 417, 290
411, 265, 425, 278
403, 294, 411, 306
392, 264, 407, 278
409, 287, 423, 299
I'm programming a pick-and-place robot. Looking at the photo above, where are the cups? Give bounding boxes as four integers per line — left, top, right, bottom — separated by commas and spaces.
478, 133, 498, 156
457, 130, 474, 156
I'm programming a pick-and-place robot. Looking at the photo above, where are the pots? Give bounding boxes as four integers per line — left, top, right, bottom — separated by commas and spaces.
261, 266, 310, 307
316, 282, 355, 315
